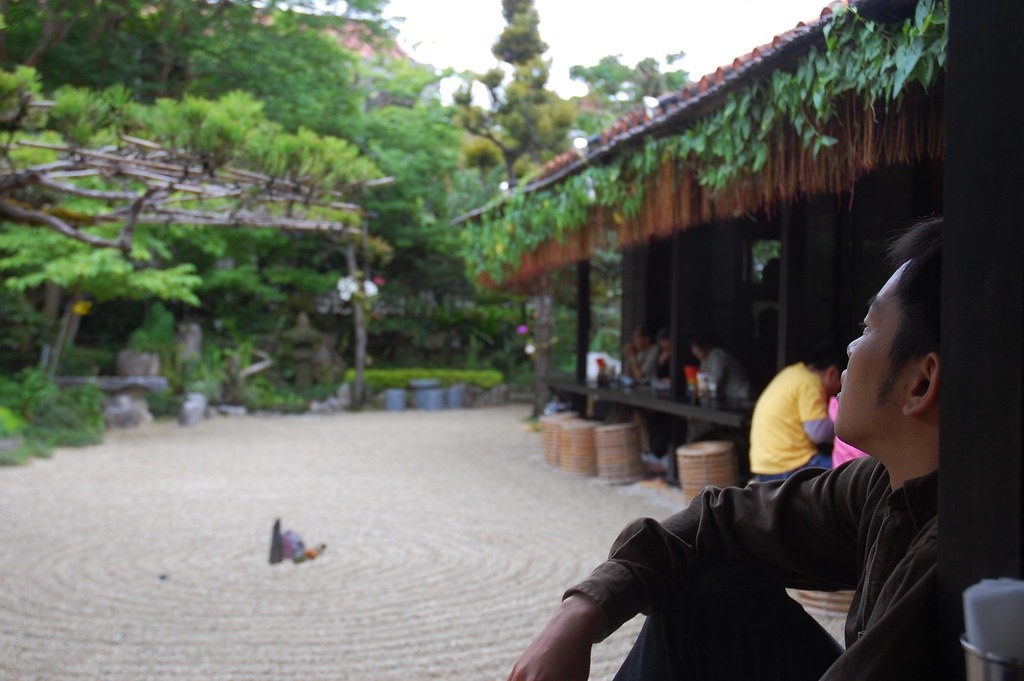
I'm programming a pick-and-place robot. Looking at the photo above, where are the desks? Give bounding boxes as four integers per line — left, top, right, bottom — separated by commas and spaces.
537, 374, 760, 486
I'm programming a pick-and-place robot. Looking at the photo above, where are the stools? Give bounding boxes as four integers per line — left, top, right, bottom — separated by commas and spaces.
541, 412, 578, 468
594, 422, 645, 487
675, 441, 738, 508
558, 420, 595, 480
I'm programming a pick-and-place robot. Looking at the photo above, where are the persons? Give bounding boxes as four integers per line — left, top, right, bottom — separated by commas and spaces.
509, 211, 944, 681
750, 335, 871, 482
608, 323, 758, 474
